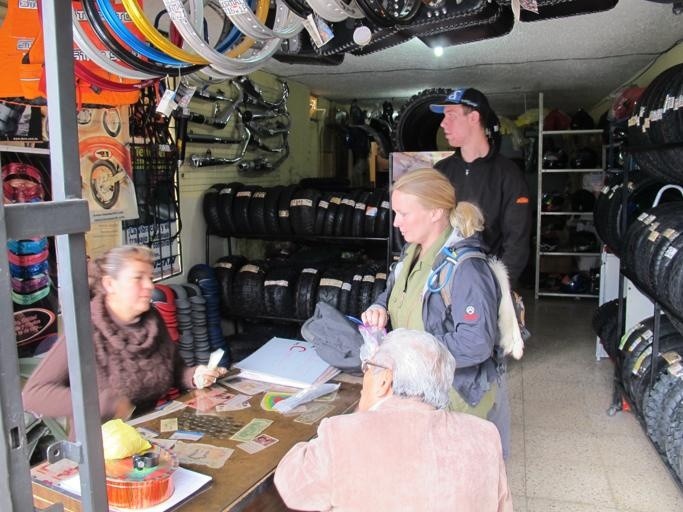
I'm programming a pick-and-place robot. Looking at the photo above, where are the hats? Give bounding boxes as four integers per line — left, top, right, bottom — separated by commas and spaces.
429, 88, 490, 114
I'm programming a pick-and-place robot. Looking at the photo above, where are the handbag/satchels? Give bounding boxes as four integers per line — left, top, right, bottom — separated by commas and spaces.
301, 302, 364, 377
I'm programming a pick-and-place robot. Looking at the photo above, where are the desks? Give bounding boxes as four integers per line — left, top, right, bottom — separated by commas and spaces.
29, 364, 365, 512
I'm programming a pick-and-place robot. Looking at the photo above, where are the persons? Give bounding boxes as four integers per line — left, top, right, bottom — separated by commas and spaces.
274, 327, 514, 512
22, 245, 227, 443
361, 169, 509, 462
430, 86, 533, 290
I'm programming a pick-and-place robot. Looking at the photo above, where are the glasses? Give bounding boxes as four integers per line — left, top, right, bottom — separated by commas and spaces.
361, 359, 387, 374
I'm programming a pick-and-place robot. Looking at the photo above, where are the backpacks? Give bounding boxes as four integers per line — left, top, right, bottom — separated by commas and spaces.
431, 231, 532, 361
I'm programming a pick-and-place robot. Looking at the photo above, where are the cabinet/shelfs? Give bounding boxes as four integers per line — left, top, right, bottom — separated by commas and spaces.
535, 94, 608, 302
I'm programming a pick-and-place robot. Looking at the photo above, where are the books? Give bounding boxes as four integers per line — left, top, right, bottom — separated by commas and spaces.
234, 336, 342, 389
